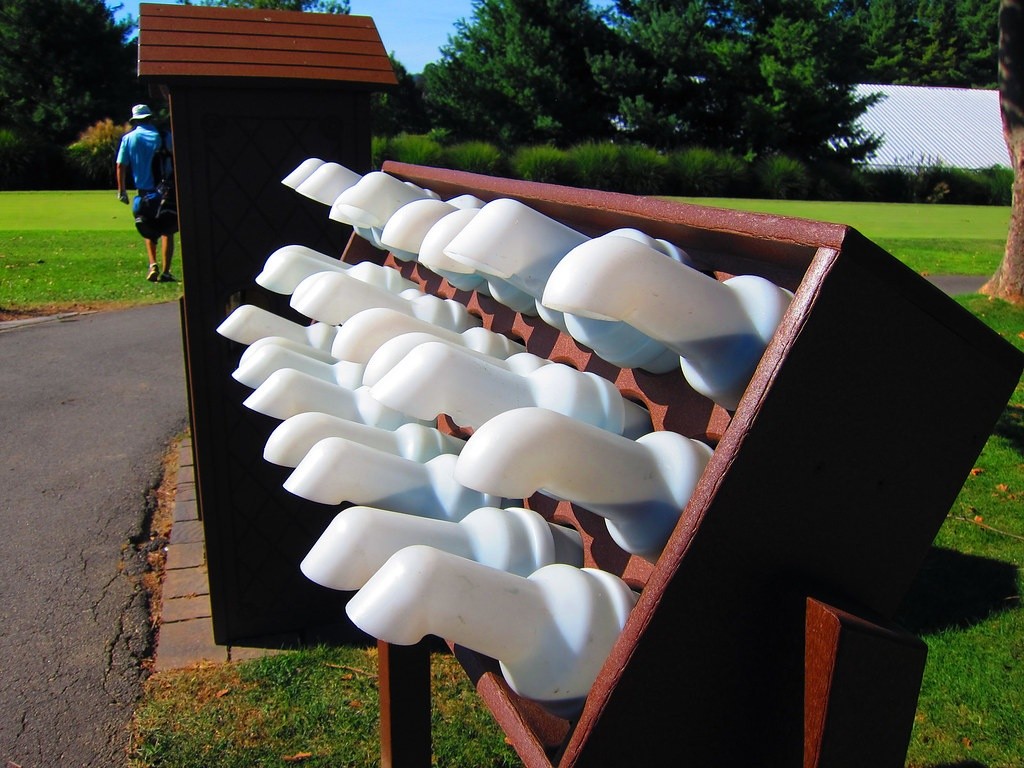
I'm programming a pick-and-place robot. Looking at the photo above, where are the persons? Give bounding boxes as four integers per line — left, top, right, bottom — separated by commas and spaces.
115, 103, 179, 283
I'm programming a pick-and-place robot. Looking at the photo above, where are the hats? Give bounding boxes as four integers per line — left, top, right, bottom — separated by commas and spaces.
129, 104, 153, 123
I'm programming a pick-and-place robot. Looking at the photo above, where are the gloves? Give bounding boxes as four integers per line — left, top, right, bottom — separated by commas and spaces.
118, 192, 129, 204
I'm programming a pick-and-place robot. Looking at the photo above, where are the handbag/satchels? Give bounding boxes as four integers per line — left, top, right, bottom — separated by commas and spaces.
133, 173, 178, 244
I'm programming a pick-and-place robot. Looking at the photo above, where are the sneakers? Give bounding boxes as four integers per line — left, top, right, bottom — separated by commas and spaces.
146, 264, 159, 281
157, 271, 176, 281
